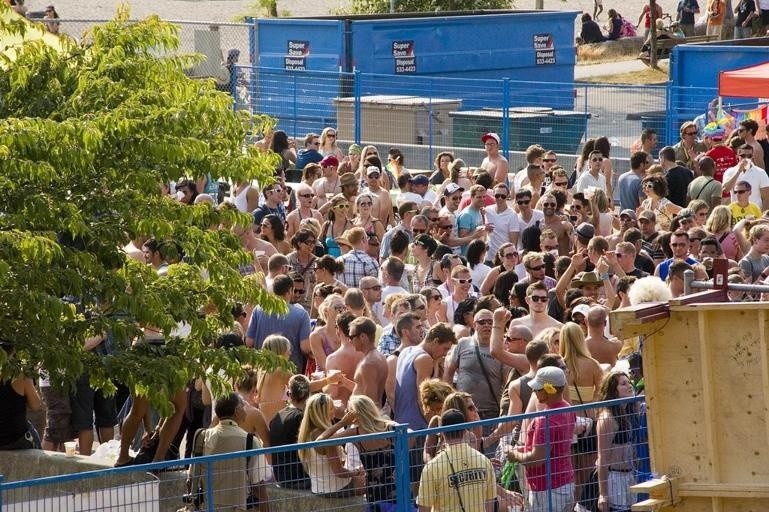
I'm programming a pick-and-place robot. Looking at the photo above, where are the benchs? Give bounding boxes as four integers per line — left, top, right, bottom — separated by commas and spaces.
637, 34, 719, 63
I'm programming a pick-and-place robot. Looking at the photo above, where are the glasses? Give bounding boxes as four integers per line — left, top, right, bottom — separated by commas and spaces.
640, 221, 653, 225
738, 153, 754, 159
554, 182, 568, 186
494, 193, 507, 200
412, 228, 426, 234
733, 189, 749, 194
517, 199, 530, 205
416, 240, 425, 247
347, 334, 358, 340
334, 306, 346, 313
368, 174, 378, 179
333, 204, 350, 209
689, 237, 702, 243
320, 165, 333, 168
279, 265, 293, 271
427, 216, 440, 222
362, 285, 382, 291
502, 335, 529, 344
645, 184, 654, 189
300, 240, 316, 246
299, 193, 315, 198
670, 242, 686, 248
310, 141, 321, 146
359, 201, 373, 206
570, 204, 582, 210
437, 223, 453, 229
542, 158, 556, 162
542, 202, 556, 208
292, 288, 307, 294
638, 160, 651, 164
526, 264, 546, 271
472, 319, 493, 326
448, 195, 463, 201
527, 295, 548, 303
464, 403, 477, 412
697, 212, 708, 216
369, 241, 380, 247
451, 277, 473, 286
366, 151, 378, 156
684, 131, 698, 135
507, 292, 522, 300
266, 187, 284, 193
428, 294, 443, 301
542, 244, 560, 251
260, 222, 272, 228
615, 252, 625, 258
414, 304, 426, 310
574, 319, 585, 326
588, 157, 603, 162
326, 134, 335, 138
619, 218, 631, 222
501, 251, 519, 258
313, 268, 321, 272
407, 208, 420, 214
737, 129, 746, 133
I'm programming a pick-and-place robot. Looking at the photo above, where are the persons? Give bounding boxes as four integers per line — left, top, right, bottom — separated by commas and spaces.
181, 393, 273, 512
459, 184, 487, 255
509, 279, 566, 340
229, 214, 291, 277
316, 395, 417, 512
245, 273, 313, 376
40, 6, 60, 38
636, 175, 684, 232
635, 0, 663, 42
394, 322, 457, 482
439, 183, 486, 256
577, 12, 606, 45
482, 185, 520, 266
592, 0, 603, 21
685, 96, 742, 141
573, 189, 767, 301
269, 375, 313, 491
670, 122, 709, 166
706, 0, 727, 40
735, 120, 765, 172
685, 156, 723, 214
428, 150, 496, 211
319, 197, 356, 259
595, 371, 641, 511
286, 185, 324, 241
504, 365, 577, 511
342, 316, 388, 437
296, 392, 368, 499
697, 122, 736, 179
577, 150, 607, 205
256, 335, 343, 428
254, 123, 390, 210
324, 310, 362, 427
115, 172, 260, 280
0, 288, 254, 472
600, 8, 622, 42
479, 131, 510, 186
616, 12, 637, 37
489, 305, 531, 441
555, 320, 603, 509
668, 22, 685, 39
533, 193, 577, 258
417, 409, 498, 512
734, 0, 756, 39
349, 196, 386, 259
509, 188, 545, 257
333, 227, 377, 290
213, 47, 248, 114
721, 145, 768, 214
727, 180, 762, 228
109, 261, 660, 370
246, 180, 286, 241
656, 145, 693, 209
380, 146, 445, 263
637, 18, 668, 67
510, 140, 595, 194
675, 0, 700, 37
445, 309, 507, 439
636, 127, 658, 171
753, 0, 769, 28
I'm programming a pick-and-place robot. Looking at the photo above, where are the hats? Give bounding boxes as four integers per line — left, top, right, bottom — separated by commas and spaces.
526, 366, 566, 391
619, 208, 636, 221
481, 133, 500, 145
334, 229, 353, 248
636, 210, 656, 224
407, 173, 428, 185
444, 182, 465, 197
571, 304, 590, 320
571, 271, 604, 289
338, 171, 360, 187
447, 158, 466, 174
366, 165, 380, 176
573, 222, 595, 238
319, 154, 340, 170
441, 408, 465, 433
536, 352, 567, 370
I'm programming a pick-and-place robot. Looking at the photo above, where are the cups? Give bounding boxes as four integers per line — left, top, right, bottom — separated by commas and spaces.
333, 399, 343, 409
312, 371, 324, 380
703, 256, 713, 271
325, 192, 334, 202
584, 189, 591, 200
175, 190, 185, 200
477, 225, 485, 242
329, 369, 340, 384
744, 158, 751, 169
255, 250, 265, 257
